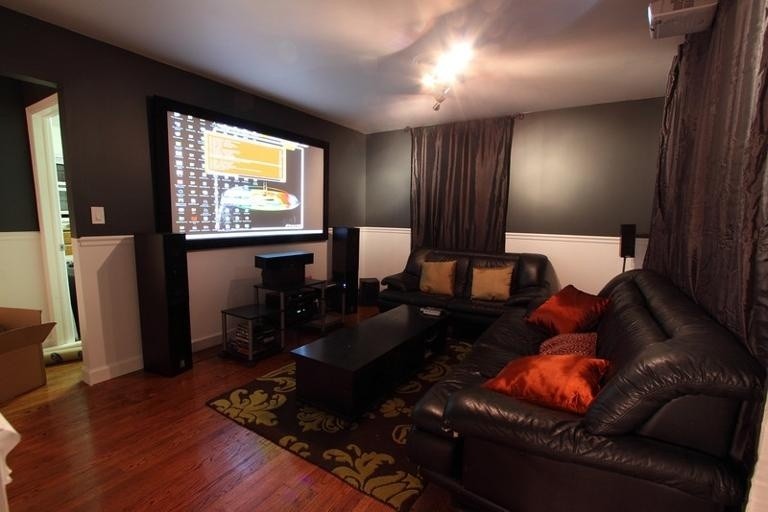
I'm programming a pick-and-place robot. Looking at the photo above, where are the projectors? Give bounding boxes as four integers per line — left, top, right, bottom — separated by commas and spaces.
648, 0, 718, 40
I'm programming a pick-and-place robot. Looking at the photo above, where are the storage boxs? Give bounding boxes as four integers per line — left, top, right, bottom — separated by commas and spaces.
0, 308, 57, 403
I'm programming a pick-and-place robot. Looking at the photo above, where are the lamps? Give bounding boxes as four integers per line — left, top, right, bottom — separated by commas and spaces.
621, 224, 636, 275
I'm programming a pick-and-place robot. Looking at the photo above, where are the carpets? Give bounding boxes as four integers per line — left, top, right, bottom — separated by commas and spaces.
206, 330, 472, 510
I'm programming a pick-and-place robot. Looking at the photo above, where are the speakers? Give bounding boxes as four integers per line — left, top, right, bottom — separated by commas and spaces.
359, 278, 380, 306
619, 223, 635, 259
326, 226, 359, 314
134, 231, 193, 377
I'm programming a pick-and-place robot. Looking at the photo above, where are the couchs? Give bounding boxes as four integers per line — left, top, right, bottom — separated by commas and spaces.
405, 269, 767, 511
377, 248, 552, 338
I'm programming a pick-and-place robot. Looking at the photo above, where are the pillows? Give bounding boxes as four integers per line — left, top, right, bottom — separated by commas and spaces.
538, 332, 597, 357
470, 265, 513, 303
479, 354, 611, 414
523, 284, 609, 336
419, 260, 457, 296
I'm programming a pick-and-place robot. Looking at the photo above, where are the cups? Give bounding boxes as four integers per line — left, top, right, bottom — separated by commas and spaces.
218, 186, 301, 230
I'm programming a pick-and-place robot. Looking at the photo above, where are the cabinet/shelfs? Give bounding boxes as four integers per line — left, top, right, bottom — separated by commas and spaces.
220, 275, 347, 369
